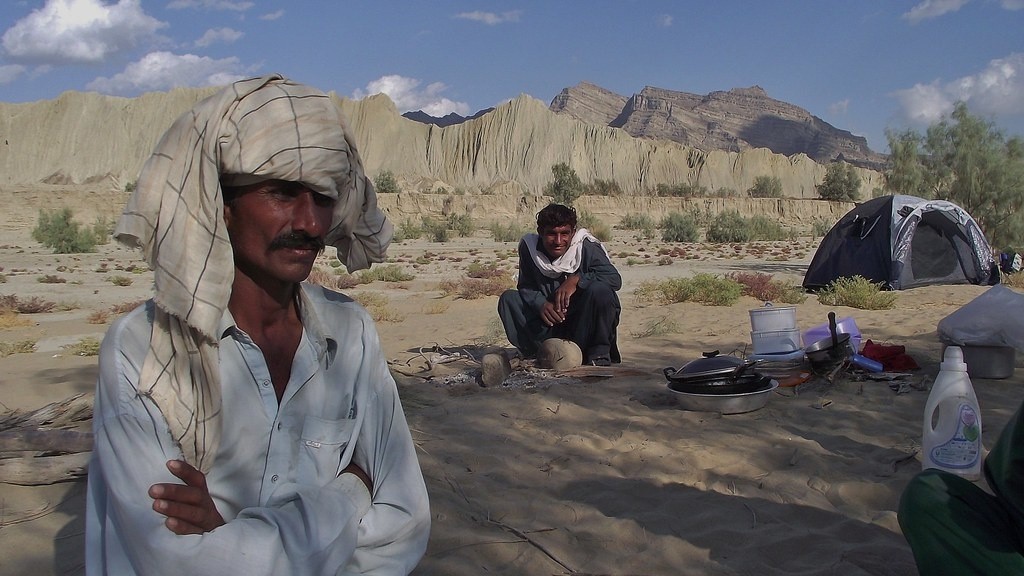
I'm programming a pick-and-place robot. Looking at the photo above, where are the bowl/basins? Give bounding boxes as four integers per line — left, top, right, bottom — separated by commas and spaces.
942, 345, 1015, 380
748, 306, 796, 332
668, 380, 779, 415
750, 329, 800, 351
805, 332, 851, 375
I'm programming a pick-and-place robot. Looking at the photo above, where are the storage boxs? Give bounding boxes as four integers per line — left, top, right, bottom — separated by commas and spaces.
749, 307, 806, 377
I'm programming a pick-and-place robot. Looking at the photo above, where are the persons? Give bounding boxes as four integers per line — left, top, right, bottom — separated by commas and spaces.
898, 403, 1024, 575
84, 76, 432, 576
498, 204, 622, 366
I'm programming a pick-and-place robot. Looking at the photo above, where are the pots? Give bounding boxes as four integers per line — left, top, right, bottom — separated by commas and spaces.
668, 374, 773, 394
663, 349, 757, 381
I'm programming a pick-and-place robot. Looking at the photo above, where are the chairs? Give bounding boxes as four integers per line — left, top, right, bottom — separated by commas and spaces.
999, 247, 1024, 286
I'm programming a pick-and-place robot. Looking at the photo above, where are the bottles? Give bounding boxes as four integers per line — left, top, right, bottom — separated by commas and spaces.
922, 346, 982, 482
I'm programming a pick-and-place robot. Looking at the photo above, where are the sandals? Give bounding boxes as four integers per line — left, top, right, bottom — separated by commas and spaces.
589, 353, 611, 366
522, 354, 537, 363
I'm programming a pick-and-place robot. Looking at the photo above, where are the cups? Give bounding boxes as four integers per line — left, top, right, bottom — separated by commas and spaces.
852, 355, 884, 373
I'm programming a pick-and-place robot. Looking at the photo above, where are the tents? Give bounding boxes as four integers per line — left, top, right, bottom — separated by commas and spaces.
801, 194, 999, 291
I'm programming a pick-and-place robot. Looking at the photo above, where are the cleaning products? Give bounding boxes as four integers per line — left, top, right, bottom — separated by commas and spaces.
921, 344, 983, 483
